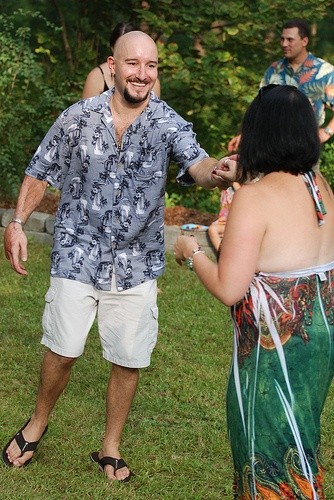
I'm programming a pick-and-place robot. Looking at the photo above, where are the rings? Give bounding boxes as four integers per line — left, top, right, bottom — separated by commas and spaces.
225, 158, 230, 166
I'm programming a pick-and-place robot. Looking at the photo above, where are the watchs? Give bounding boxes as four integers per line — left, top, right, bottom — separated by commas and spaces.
8, 217, 26, 226
185, 249, 205, 270
324, 127, 333, 136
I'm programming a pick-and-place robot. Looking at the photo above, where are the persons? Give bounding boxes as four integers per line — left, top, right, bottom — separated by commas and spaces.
227, 21, 334, 156
174, 84, 332, 500
83, 23, 160, 100
4, 31, 239, 483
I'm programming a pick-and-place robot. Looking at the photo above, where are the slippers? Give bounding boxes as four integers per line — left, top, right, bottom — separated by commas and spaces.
2, 418, 48, 468
90, 452, 131, 483
